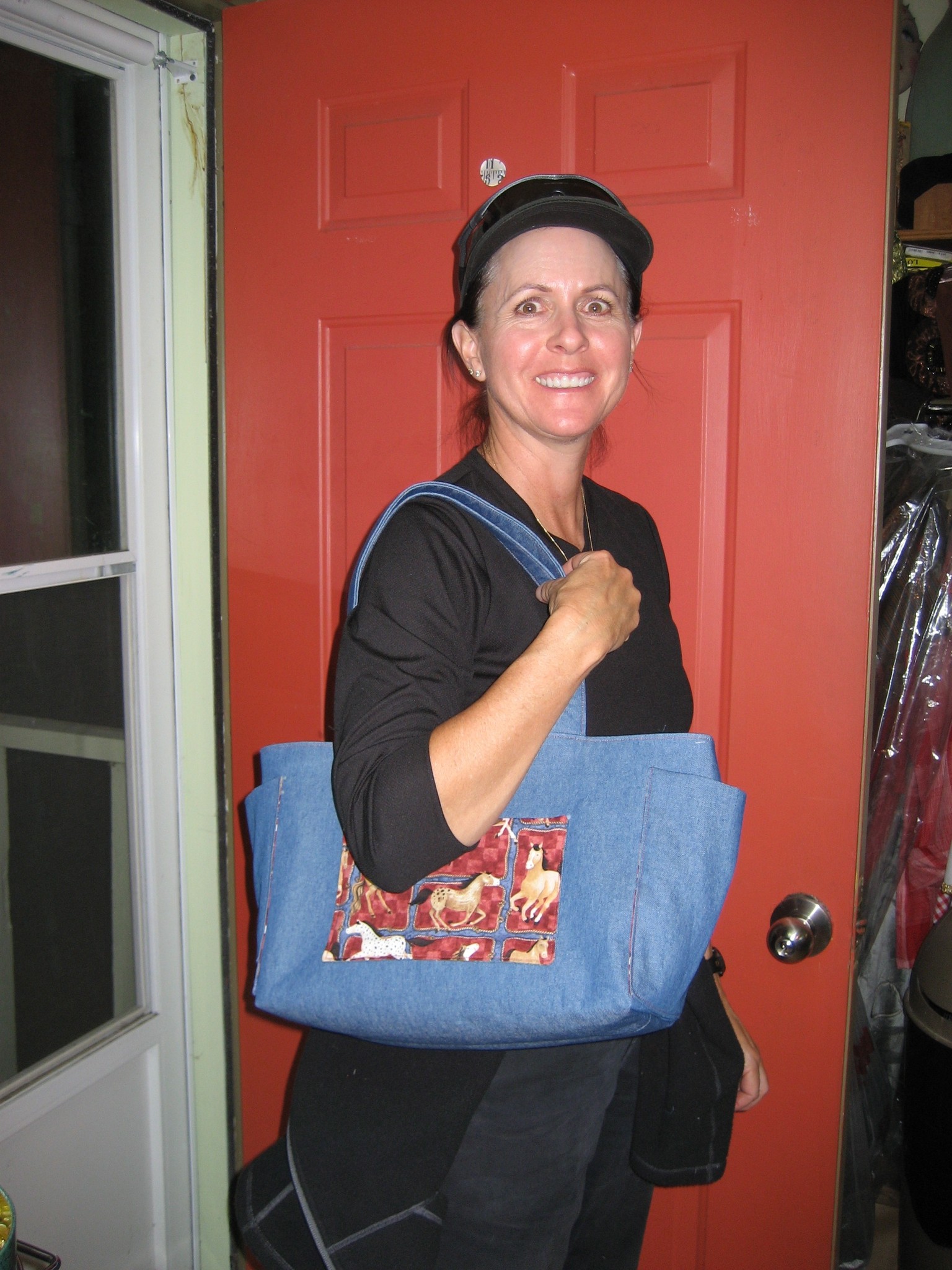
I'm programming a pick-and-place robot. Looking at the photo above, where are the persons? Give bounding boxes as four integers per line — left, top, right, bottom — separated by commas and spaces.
226, 173, 771, 1270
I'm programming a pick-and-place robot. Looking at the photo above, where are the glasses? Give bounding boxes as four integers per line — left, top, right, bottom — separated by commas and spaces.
460, 174, 623, 282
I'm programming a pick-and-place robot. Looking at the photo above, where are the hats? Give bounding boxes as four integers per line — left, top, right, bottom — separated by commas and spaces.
455, 184, 654, 316
897, 183, 952, 242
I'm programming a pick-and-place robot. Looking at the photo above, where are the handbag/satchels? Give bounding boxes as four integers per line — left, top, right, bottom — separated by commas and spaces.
236, 480, 749, 1050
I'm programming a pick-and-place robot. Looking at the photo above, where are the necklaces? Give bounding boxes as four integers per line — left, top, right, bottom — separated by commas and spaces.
480, 437, 594, 561
